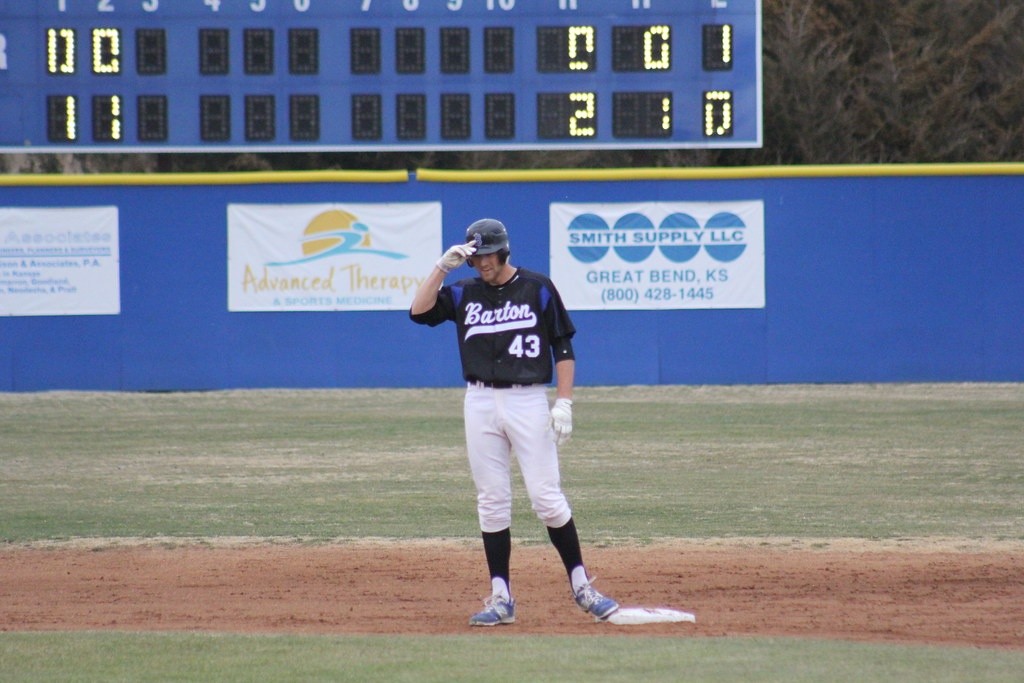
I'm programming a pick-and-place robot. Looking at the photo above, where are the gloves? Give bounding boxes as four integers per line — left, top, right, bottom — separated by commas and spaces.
547, 399, 573, 446
436, 240, 477, 274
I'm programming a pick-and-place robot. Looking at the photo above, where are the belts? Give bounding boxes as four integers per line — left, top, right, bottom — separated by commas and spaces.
467, 377, 533, 389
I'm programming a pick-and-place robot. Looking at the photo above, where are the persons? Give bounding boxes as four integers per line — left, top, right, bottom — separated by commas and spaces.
408, 218, 619, 627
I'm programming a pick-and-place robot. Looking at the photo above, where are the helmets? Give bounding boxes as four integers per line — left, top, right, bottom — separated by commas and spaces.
466, 218, 510, 267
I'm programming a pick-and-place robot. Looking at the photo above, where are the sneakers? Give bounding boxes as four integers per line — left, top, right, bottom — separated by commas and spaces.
469, 592, 514, 627
572, 575, 619, 620
